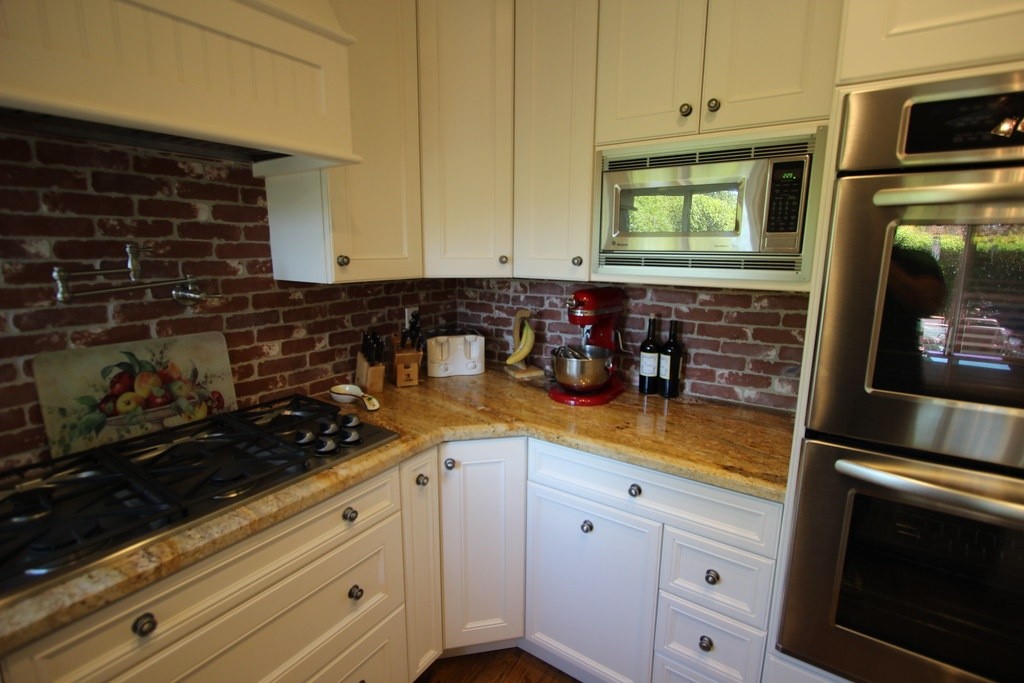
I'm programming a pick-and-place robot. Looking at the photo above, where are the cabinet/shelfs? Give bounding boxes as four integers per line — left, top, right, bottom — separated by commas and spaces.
439, 436, 528, 649
597, 0, 841, 145
399, 446, 442, 683
513, 0, 597, 282
264, 0, 422, 281
0, 464, 409, 683
416, 0, 514, 278
525, 437, 785, 683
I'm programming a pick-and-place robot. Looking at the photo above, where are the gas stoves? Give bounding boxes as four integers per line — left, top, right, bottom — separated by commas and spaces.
1, 393, 399, 610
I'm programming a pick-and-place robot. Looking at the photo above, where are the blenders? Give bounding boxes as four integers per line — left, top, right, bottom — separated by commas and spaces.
548, 287, 634, 406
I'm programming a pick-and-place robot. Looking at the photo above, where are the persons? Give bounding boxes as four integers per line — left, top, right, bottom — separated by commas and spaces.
872, 242, 947, 398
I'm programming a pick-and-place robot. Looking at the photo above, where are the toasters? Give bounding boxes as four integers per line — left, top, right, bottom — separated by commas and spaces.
426, 327, 486, 377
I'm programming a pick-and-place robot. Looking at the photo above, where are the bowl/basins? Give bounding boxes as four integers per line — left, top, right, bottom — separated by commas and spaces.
331, 384, 380, 410
550, 344, 614, 394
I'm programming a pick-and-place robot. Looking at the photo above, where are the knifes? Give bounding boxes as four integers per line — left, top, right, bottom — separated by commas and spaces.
398, 311, 424, 352
361, 330, 386, 366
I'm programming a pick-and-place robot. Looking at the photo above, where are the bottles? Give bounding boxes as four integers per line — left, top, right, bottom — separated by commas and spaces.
660, 320, 683, 399
638, 318, 660, 395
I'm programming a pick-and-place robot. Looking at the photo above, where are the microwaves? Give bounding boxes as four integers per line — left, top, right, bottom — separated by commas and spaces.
589, 119, 830, 285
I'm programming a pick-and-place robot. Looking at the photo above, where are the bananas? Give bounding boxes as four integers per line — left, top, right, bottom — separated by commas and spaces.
506, 319, 535, 364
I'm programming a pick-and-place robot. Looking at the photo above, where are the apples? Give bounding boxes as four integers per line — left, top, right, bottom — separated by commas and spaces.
99, 363, 224, 422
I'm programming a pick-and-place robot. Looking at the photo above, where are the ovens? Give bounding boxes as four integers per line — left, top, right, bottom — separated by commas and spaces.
774, 69, 1024, 683
